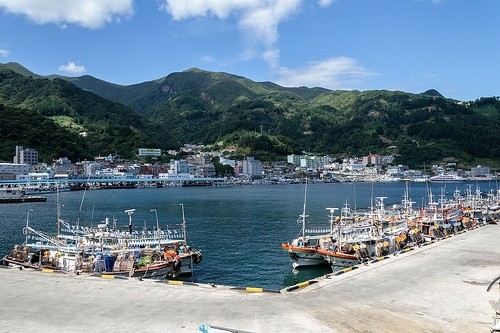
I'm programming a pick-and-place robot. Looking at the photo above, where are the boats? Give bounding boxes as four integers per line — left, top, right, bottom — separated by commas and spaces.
280, 172, 500, 273
1, 184, 203, 279
1, 186, 48, 204
